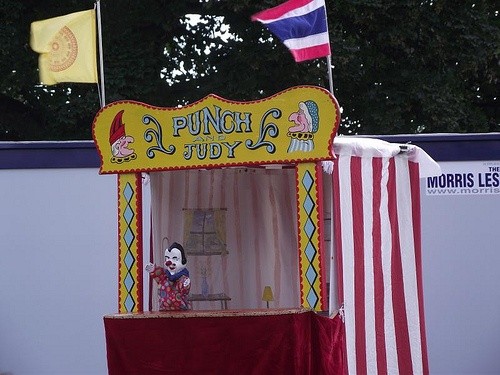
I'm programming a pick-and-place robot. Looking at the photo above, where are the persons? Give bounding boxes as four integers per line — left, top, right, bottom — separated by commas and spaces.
145, 243, 193, 311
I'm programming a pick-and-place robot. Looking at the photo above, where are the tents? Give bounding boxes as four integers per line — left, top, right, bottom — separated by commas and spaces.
142, 135, 442, 375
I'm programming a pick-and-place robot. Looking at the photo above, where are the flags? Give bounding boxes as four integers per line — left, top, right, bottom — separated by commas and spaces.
251, 0, 330, 63
30, 9, 98, 86
